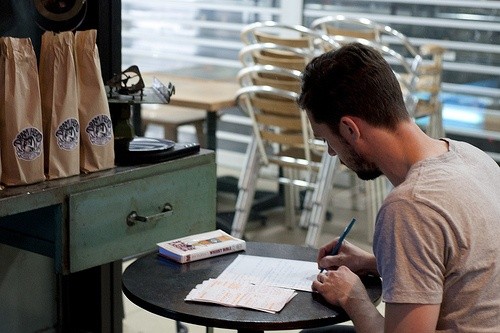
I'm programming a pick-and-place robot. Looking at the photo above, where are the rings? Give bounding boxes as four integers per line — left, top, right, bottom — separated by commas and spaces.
316, 272, 329, 283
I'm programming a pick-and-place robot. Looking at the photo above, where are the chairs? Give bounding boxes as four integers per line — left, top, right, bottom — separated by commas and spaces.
231, 14, 444, 248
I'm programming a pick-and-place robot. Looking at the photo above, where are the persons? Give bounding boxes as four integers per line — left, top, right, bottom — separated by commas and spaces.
295, 42, 497, 332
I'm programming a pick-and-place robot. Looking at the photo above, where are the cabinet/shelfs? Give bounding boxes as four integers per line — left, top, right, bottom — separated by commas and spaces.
0, 150, 217, 332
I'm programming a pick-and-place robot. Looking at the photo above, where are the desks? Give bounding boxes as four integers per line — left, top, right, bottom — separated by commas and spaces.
121, 241, 382, 333
137, 73, 286, 225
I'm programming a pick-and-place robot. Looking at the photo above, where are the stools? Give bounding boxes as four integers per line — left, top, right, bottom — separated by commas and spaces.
140, 103, 207, 149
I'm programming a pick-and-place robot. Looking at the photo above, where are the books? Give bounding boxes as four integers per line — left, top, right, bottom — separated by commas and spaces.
154, 227, 249, 266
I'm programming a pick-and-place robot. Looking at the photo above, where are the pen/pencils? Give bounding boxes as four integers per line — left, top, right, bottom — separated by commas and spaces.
319, 218, 356, 274
